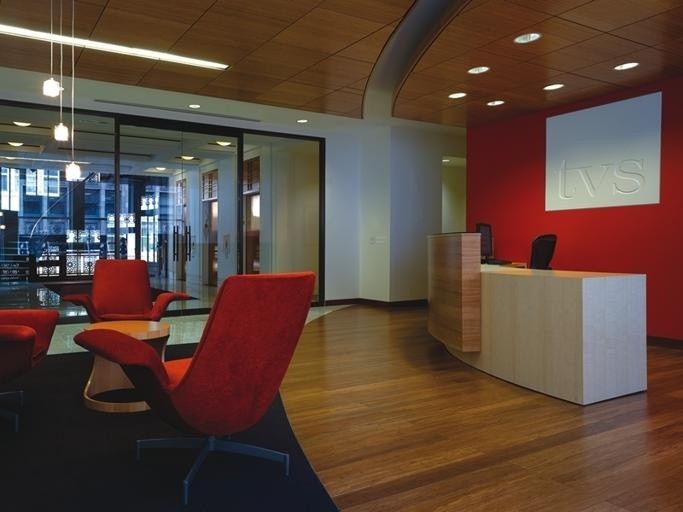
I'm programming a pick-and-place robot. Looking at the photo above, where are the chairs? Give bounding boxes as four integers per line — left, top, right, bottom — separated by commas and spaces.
529, 234, 557, 270
58, 259, 191, 322
0, 309, 61, 428
69, 272, 319, 507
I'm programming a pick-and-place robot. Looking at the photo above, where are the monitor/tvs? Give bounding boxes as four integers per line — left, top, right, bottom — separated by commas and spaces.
476, 223, 493, 257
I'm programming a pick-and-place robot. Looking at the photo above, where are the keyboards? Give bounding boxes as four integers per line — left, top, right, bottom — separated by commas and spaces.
484, 259, 512, 265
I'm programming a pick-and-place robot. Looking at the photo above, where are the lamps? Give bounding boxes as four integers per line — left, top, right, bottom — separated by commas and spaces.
43, 0, 59, 97
54, 0, 69, 141
64, 0, 82, 180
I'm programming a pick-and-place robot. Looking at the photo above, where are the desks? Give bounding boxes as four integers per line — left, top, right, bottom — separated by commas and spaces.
81, 320, 169, 413
